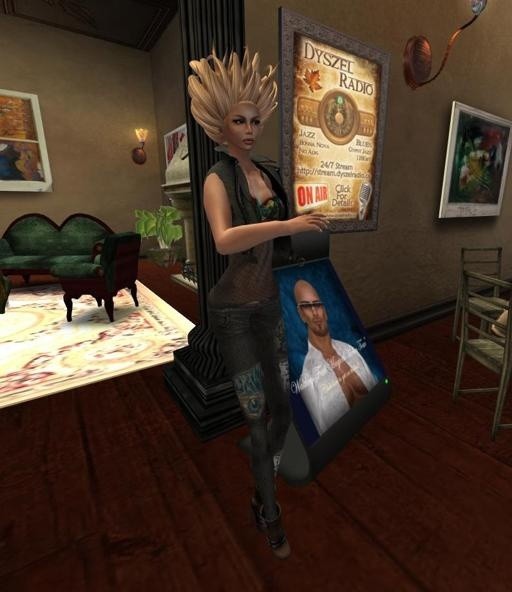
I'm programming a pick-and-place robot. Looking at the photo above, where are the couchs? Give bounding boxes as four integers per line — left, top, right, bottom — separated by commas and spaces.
0, 214, 111, 313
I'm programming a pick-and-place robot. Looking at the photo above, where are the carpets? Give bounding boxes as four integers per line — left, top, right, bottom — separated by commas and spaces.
0, 276, 195, 409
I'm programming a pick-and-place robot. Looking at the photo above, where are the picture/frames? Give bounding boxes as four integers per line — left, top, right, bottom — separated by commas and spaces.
0, 89, 51, 195
162, 122, 192, 186
434, 99, 512, 223
278, 9, 392, 237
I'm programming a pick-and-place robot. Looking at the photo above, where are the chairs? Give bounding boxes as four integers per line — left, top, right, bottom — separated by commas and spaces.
452, 244, 512, 437
61, 232, 141, 323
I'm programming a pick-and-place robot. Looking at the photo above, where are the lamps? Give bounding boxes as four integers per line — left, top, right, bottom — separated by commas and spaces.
132, 128, 149, 164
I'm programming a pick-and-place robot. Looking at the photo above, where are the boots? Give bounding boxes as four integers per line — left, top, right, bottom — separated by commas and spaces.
249, 493, 289, 559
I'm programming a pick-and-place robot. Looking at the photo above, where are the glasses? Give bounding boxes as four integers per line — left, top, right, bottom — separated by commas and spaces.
299, 302, 325, 310
